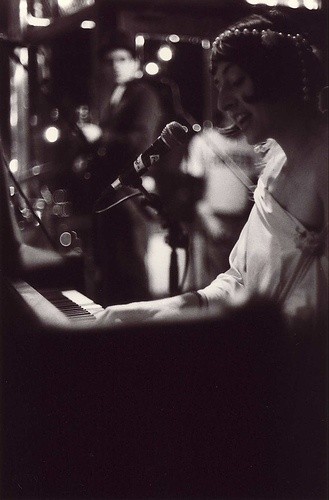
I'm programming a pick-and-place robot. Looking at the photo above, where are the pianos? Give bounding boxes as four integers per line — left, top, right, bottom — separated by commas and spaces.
0, 154, 123, 329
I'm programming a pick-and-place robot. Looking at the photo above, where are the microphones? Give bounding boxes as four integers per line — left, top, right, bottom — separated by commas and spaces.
94, 121, 186, 209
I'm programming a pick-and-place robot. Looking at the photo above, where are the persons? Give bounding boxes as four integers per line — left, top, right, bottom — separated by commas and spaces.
178, 105, 270, 294
77, 38, 161, 309
93, 14, 328, 329
